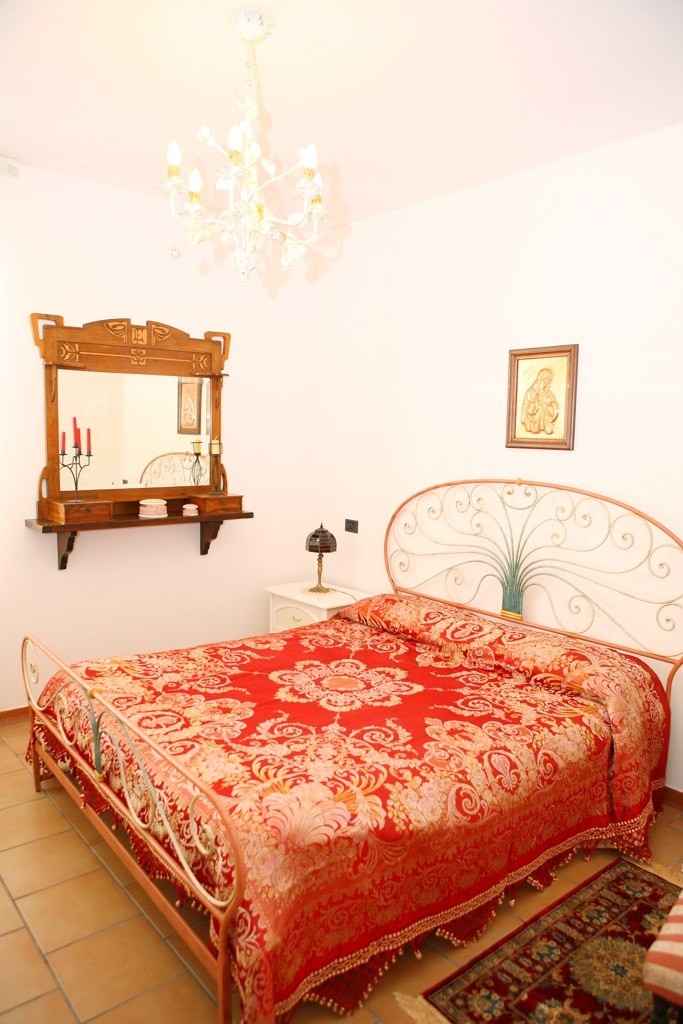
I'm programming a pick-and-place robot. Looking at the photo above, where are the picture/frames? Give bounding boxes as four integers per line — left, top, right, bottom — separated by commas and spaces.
506, 344, 580, 451
177, 379, 202, 434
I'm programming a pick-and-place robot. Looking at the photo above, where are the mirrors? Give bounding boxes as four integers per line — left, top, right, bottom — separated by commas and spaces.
57, 368, 212, 492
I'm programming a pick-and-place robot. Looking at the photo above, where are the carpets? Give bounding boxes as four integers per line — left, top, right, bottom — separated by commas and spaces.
392, 854, 683, 1024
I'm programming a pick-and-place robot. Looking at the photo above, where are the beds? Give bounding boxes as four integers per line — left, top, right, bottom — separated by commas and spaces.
21, 477, 683, 1024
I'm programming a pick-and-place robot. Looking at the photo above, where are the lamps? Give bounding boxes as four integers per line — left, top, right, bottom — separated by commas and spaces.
306, 522, 336, 592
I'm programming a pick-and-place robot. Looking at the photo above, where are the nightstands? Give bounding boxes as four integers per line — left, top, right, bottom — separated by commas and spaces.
267, 580, 373, 633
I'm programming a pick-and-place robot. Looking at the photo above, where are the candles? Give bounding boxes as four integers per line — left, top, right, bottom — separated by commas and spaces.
60, 418, 94, 452
194, 438, 201, 453
212, 434, 220, 456
